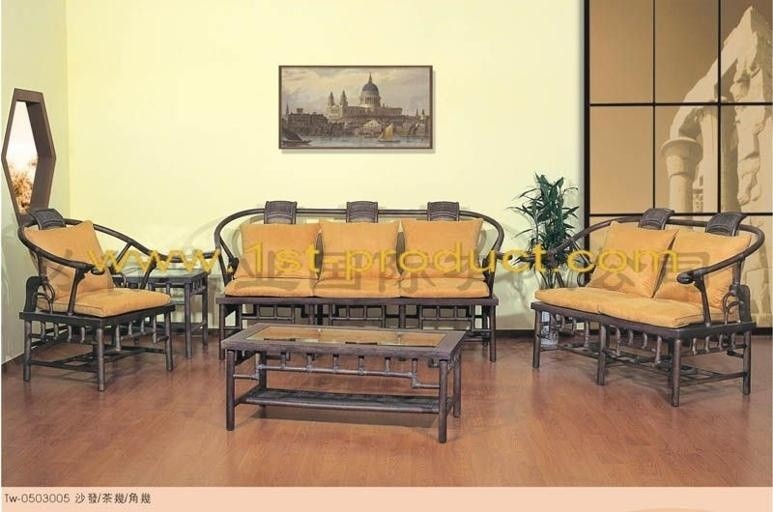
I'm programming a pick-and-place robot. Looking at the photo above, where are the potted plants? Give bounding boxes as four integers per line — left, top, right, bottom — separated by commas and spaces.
506, 171, 583, 345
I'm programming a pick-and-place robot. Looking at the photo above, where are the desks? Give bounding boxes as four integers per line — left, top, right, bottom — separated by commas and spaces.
110, 265, 209, 359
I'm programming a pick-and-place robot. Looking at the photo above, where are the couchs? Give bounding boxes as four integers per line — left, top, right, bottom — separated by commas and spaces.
518, 208, 765, 407
17, 208, 176, 391
214, 201, 505, 363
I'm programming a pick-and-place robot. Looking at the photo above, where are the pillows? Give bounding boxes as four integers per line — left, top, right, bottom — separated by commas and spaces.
584, 219, 677, 299
399, 215, 490, 283
314, 217, 404, 284
654, 226, 752, 313
23, 219, 115, 295
230, 219, 323, 280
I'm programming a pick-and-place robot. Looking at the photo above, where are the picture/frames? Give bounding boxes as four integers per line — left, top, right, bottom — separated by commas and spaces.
583, 0, 768, 336
279, 65, 432, 149
